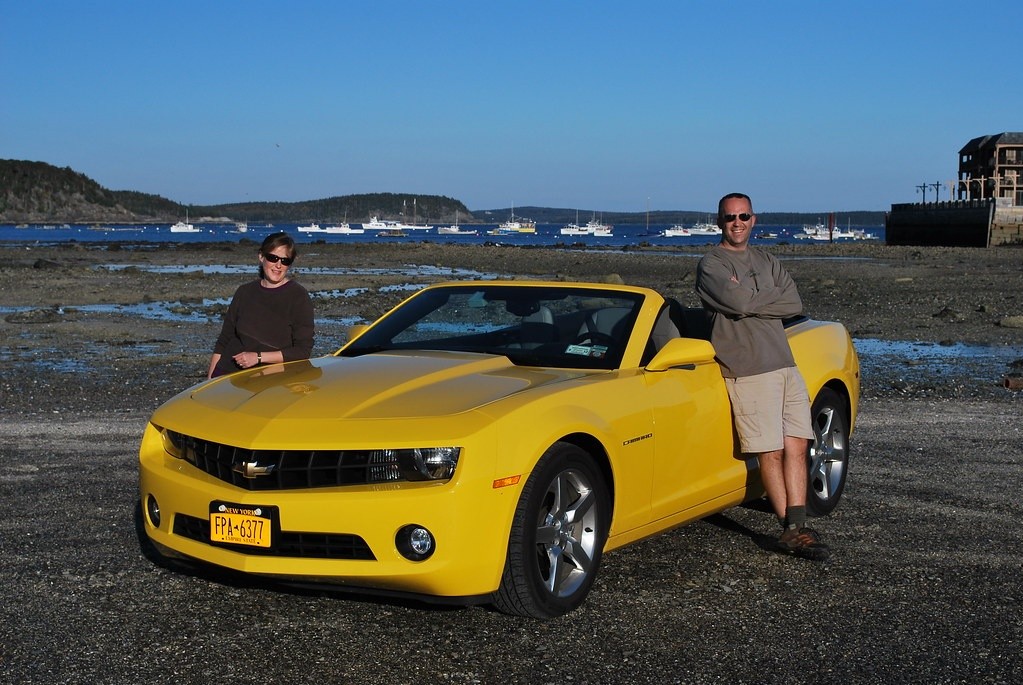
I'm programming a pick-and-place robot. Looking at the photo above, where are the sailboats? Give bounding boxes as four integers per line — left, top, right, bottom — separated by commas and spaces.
396, 198, 434, 229
637, 197, 662, 238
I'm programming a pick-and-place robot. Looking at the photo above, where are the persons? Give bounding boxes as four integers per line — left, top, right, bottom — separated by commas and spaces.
230, 360, 322, 394
694, 193, 831, 562
207, 233, 315, 380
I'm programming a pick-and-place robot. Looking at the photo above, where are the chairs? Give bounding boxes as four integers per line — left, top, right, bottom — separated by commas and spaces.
577, 308, 682, 353
502, 305, 561, 349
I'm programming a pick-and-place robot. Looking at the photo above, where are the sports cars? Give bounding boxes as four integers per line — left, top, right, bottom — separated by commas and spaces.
138, 281, 858, 620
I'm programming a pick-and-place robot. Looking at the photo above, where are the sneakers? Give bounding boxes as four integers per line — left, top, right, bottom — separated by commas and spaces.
776, 521, 830, 559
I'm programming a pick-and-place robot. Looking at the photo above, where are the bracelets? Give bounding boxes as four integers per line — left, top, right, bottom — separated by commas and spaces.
259, 371, 264, 376
257, 351, 261, 363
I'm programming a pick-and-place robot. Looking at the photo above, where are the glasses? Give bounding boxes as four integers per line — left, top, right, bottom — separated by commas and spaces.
263, 252, 293, 266
722, 213, 752, 222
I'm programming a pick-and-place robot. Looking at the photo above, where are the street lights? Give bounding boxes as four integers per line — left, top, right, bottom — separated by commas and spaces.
916, 172, 1020, 207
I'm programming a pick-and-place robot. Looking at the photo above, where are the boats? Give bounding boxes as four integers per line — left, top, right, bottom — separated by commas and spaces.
170, 208, 200, 233
326, 212, 364, 234
561, 209, 613, 236
297, 223, 326, 232
362, 216, 401, 230
664, 213, 722, 237
238, 222, 247, 233
438, 209, 477, 235
378, 227, 409, 237
793, 215, 879, 241
487, 201, 536, 236
758, 232, 777, 238
